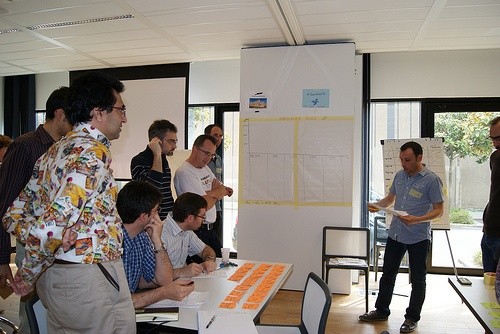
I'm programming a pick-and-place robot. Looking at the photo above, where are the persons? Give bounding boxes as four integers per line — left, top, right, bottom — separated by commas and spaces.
359, 142, 445, 333
116, 180, 195, 310
0, 134, 14, 162
0, 86, 77, 289
2, 73, 137, 334
480, 116, 500, 273
204, 125, 233, 238
139, 192, 217, 290
174, 135, 229, 264
131, 120, 177, 221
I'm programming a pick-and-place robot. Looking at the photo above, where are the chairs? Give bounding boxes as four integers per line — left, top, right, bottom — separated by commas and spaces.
373, 216, 411, 284
322, 226, 370, 313
255, 274, 333, 334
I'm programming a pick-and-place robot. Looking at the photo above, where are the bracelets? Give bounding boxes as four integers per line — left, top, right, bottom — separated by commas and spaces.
206, 256, 216, 262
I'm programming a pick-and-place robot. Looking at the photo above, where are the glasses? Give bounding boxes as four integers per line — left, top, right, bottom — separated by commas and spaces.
165, 139, 178, 145
192, 213, 207, 221
106, 104, 126, 114
486, 135, 500, 143
214, 134, 224, 140
198, 148, 216, 160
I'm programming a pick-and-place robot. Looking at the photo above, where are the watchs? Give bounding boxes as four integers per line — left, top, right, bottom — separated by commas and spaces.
153, 243, 167, 253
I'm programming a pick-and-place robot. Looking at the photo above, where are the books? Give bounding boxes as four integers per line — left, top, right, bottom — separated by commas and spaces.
135, 307, 179, 322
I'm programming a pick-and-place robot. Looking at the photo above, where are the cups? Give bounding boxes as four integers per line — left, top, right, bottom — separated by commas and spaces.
221, 248, 230, 261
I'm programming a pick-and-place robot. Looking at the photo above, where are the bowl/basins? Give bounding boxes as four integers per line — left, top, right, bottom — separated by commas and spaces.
484, 273, 495, 285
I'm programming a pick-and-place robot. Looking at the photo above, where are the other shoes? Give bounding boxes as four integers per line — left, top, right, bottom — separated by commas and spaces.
400, 318, 418, 332
359, 310, 388, 321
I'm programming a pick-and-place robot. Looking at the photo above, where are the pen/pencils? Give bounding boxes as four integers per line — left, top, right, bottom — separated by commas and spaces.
206, 315, 216, 328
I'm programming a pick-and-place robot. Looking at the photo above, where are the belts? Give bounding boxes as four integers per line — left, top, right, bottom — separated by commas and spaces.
199, 224, 214, 230
52, 258, 77, 265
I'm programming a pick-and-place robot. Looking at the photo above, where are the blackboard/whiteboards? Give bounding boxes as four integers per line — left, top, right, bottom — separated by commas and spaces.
380, 137, 451, 230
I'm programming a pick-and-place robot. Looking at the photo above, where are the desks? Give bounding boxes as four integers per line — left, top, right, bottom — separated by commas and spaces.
449, 277, 500, 334
135, 258, 294, 334
